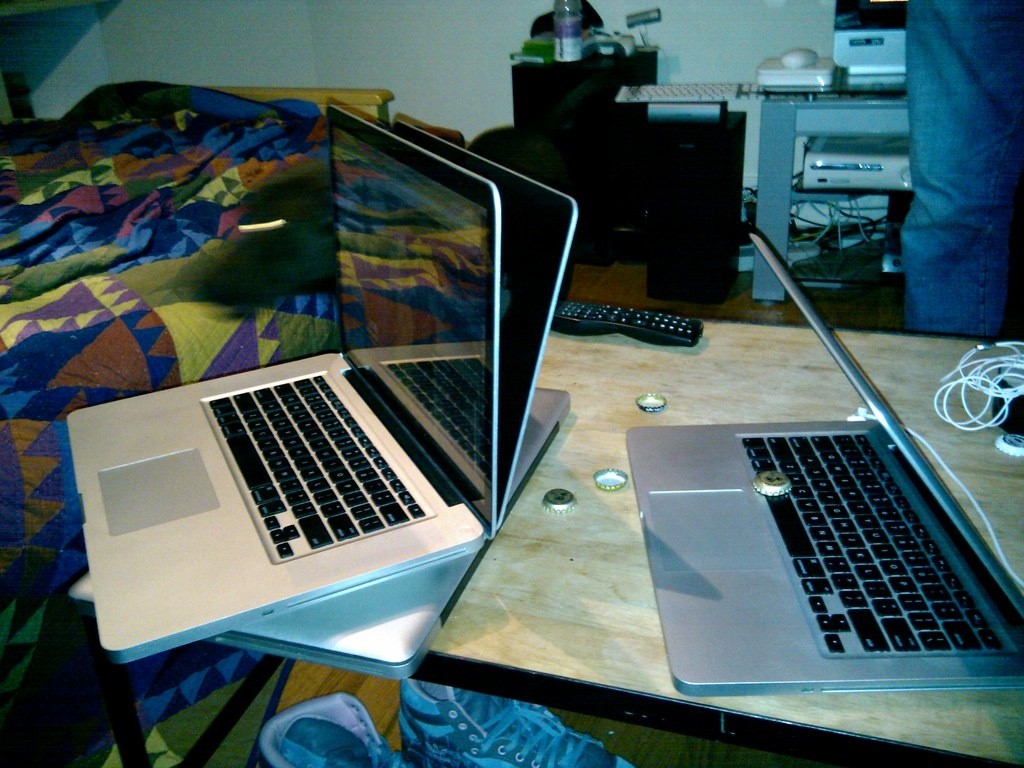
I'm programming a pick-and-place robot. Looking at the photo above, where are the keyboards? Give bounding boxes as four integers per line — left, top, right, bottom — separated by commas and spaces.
615, 85, 741, 102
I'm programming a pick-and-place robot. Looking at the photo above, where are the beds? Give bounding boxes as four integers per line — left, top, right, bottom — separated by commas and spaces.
0, 80, 396, 768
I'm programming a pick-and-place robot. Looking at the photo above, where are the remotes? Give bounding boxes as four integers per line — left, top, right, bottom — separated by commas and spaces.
551, 302, 704, 347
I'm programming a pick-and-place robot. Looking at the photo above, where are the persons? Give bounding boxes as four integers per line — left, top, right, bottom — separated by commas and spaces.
902, 0, 1024, 342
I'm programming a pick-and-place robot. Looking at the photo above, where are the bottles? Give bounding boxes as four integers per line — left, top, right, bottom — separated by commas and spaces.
553, 0, 582, 62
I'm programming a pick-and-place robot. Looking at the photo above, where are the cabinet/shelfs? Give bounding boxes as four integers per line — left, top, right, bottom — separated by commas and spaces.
509, 48, 747, 306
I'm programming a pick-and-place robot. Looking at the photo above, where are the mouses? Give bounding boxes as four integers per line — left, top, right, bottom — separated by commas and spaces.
780, 49, 818, 70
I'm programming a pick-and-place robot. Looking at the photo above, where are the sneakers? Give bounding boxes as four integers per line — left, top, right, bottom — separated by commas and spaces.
397, 677, 636, 768
258, 693, 411, 766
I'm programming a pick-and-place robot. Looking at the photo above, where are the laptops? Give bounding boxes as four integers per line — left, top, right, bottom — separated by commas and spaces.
625, 233, 1024, 696
70, 105, 579, 678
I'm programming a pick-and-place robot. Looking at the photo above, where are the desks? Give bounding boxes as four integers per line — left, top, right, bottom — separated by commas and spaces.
67, 320, 1024, 768
752, 91, 914, 305
